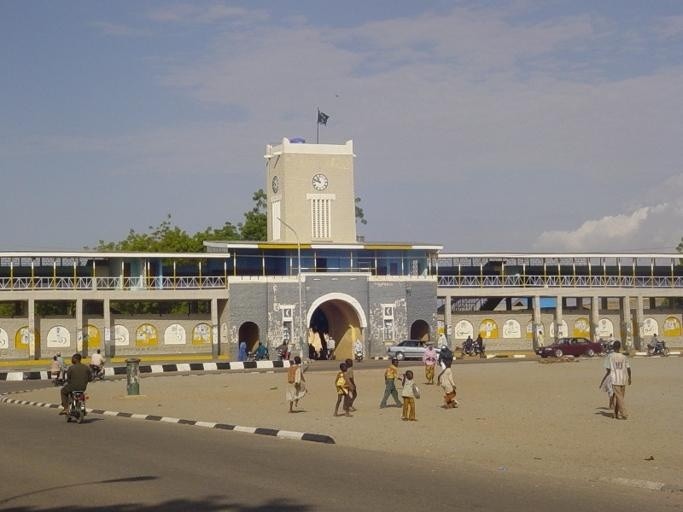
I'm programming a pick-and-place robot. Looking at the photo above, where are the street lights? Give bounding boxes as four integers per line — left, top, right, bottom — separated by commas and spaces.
272, 216, 305, 366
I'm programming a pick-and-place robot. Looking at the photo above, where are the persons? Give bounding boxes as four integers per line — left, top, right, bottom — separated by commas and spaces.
439, 359, 456, 409
437, 334, 447, 346
274, 340, 288, 359
537, 330, 543, 347
285, 356, 305, 414
55, 351, 63, 371
604, 372, 615, 409
331, 362, 353, 417
422, 345, 437, 385
465, 336, 472, 352
251, 341, 269, 361
52, 356, 58, 371
400, 371, 419, 421
607, 334, 613, 344
437, 345, 453, 369
59, 354, 91, 415
598, 340, 631, 420
237, 337, 248, 362
475, 334, 484, 355
652, 333, 660, 354
89, 349, 106, 376
308, 336, 362, 360
379, 359, 402, 408
343, 359, 357, 411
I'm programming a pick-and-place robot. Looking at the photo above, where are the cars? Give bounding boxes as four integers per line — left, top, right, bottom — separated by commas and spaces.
385, 339, 440, 362
534, 337, 601, 356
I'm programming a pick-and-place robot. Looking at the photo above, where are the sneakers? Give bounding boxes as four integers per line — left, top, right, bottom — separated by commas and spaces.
609, 402, 627, 420
333, 401, 459, 421
59, 410, 68, 415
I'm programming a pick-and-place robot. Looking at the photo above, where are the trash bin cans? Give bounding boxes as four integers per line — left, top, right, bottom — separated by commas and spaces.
125, 358, 140, 395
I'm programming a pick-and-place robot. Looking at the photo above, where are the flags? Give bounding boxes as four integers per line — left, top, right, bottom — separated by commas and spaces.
317, 111, 329, 126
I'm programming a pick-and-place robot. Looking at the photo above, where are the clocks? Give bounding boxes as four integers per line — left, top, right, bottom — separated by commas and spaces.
311, 173, 328, 191
271, 175, 279, 193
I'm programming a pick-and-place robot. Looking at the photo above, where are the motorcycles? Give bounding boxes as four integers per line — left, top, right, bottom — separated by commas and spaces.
599, 338, 617, 356
275, 348, 288, 361
461, 342, 484, 355
57, 379, 88, 424
648, 340, 669, 357
355, 350, 363, 361
323, 348, 333, 360
88, 361, 107, 382
247, 350, 269, 361
52, 369, 68, 386
